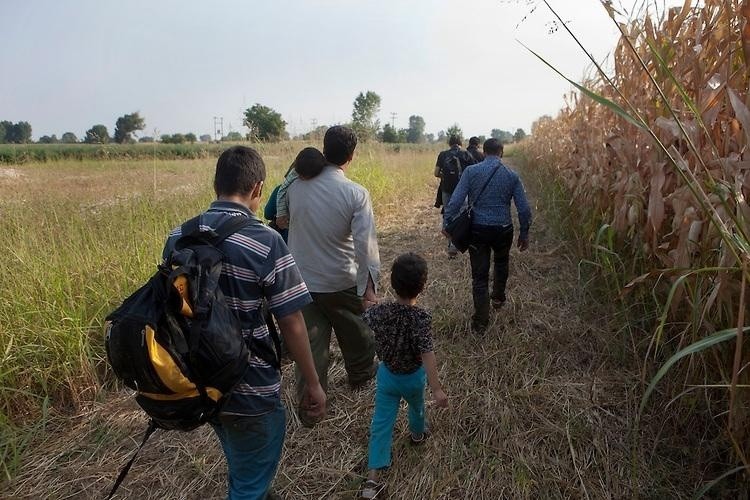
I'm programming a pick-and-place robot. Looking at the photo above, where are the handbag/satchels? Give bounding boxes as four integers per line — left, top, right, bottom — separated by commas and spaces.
445, 162, 502, 254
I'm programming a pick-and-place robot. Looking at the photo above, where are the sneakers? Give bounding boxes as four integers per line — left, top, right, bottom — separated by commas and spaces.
361, 480, 380, 500
410, 432, 427, 444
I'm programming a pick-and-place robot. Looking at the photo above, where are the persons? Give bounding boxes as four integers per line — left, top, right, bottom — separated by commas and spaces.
275, 147, 324, 229
284, 126, 381, 429
441, 139, 532, 327
465, 137, 488, 162
162, 145, 327, 500
434, 133, 480, 257
362, 253, 448, 500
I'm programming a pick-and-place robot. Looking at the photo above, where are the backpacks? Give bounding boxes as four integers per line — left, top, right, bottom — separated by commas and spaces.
102, 213, 264, 500
442, 148, 465, 194
